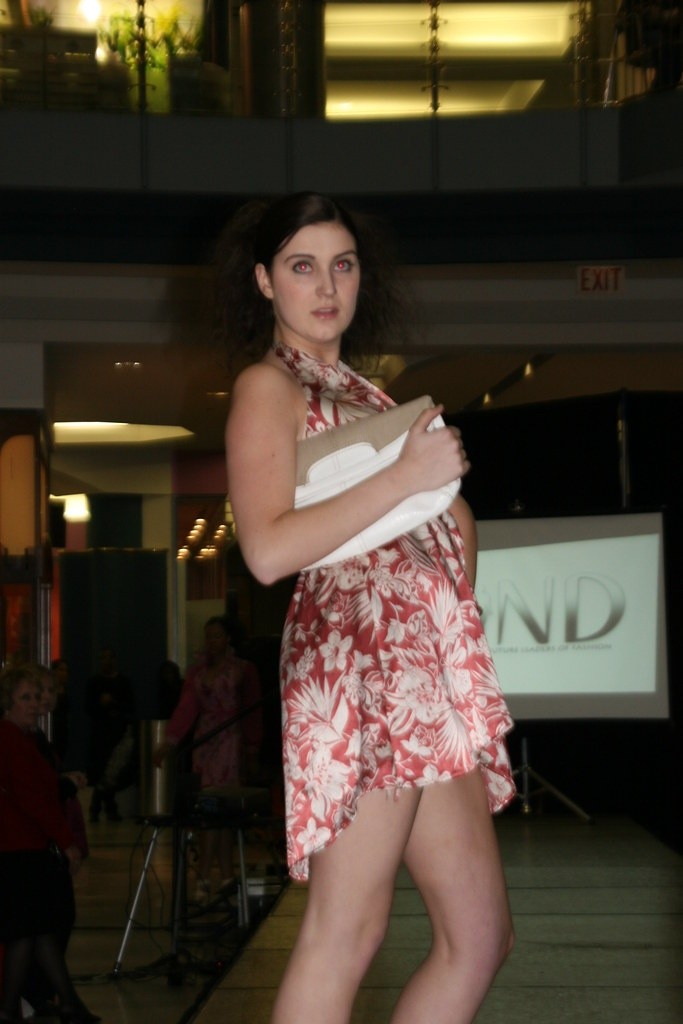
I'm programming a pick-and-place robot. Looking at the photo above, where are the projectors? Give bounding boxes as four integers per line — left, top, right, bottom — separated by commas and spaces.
195, 782, 270, 818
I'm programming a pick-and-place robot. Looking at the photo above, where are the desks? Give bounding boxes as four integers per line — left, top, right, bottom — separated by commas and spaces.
112, 812, 257, 977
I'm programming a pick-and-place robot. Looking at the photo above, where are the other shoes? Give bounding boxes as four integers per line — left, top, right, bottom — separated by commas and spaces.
106, 810, 124, 822
196, 876, 212, 902
89, 807, 101, 822
59, 1004, 103, 1024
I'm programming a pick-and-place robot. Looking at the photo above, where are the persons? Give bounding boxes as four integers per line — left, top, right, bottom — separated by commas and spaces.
153, 616, 263, 899
220, 193, 518, 1024
0, 661, 102, 1024
